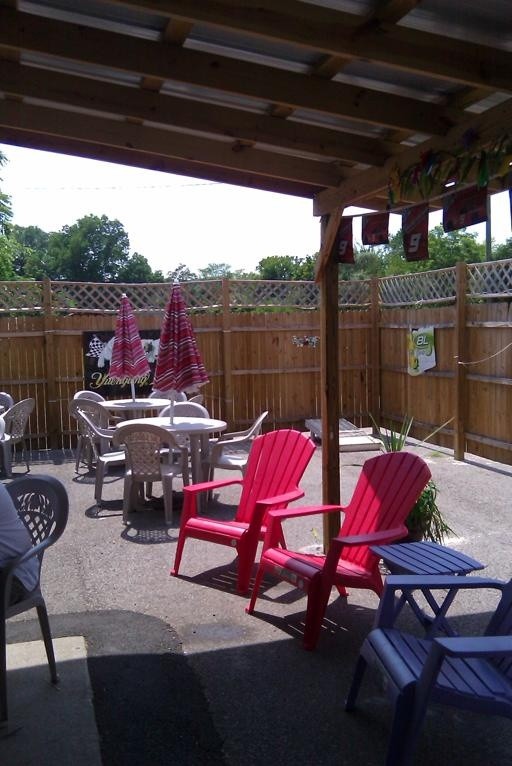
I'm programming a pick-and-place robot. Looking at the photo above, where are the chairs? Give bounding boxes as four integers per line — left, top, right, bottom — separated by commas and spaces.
245, 451, 433, 647
171, 430, 316, 594
202, 411, 269, 512
112, 424, 189, 525
67, 387, 210, 504
0, 474, 68, 734
0, 391, 36, 476
344, 575, 512, 765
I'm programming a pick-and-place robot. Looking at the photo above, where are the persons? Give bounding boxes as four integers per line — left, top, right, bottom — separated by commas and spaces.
0, 482, 41, 608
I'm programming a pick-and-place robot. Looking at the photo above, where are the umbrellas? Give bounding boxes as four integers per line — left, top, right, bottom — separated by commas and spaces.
107, 293, 149, 404
151, 277, 210, 425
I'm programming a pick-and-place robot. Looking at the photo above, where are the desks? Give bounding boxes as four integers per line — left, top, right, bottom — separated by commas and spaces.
115, 416, 228, 513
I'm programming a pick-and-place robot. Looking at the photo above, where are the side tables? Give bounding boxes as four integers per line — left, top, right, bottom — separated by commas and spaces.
368, 541, 483, 640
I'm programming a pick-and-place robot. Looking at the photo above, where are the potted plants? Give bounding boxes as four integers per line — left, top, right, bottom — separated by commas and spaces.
367, 410, 459, 568
